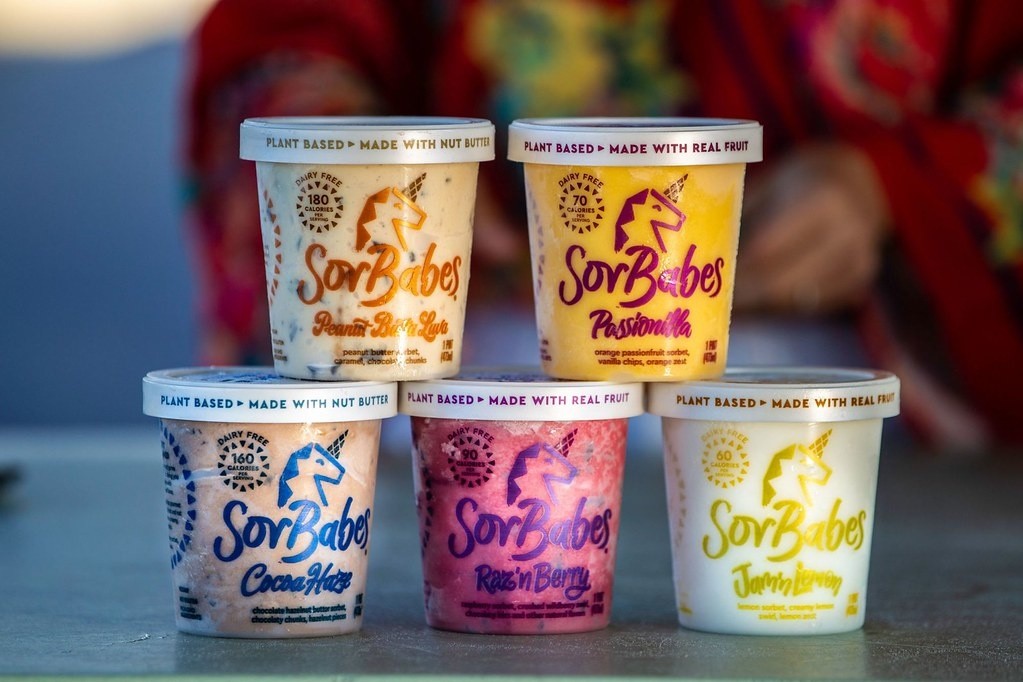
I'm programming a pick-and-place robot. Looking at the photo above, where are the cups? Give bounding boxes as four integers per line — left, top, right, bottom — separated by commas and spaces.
648, 369, 901, 636
141, 366, 399, 637
507, 116, 764, 384
398, 374, 646, 632
241, 115, 497, 383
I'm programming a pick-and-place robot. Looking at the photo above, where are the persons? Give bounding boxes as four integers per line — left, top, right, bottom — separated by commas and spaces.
177, 0, 1023, 459
